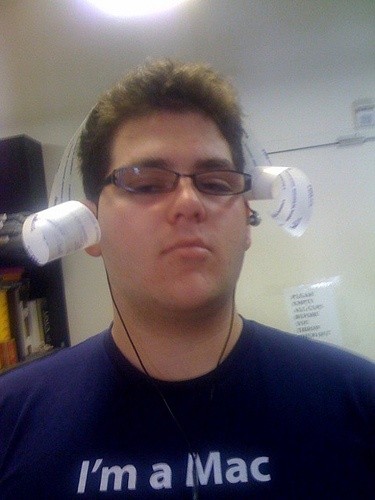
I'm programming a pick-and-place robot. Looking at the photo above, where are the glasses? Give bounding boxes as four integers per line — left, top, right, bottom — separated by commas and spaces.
91, 165, 251, 196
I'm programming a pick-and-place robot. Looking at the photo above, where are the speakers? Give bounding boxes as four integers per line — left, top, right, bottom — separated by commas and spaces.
0, 134, 50, 217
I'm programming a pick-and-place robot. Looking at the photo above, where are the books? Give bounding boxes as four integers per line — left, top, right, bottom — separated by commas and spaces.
0, 267, 56, 368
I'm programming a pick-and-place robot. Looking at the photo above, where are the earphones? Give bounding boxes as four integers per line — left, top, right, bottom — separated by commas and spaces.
248, 210, 261, 226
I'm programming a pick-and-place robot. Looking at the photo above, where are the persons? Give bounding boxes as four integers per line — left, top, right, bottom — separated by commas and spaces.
0, 59, 375, 500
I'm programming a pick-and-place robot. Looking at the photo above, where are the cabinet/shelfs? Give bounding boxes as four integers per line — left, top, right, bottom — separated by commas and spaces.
0, 134, 71, 377
